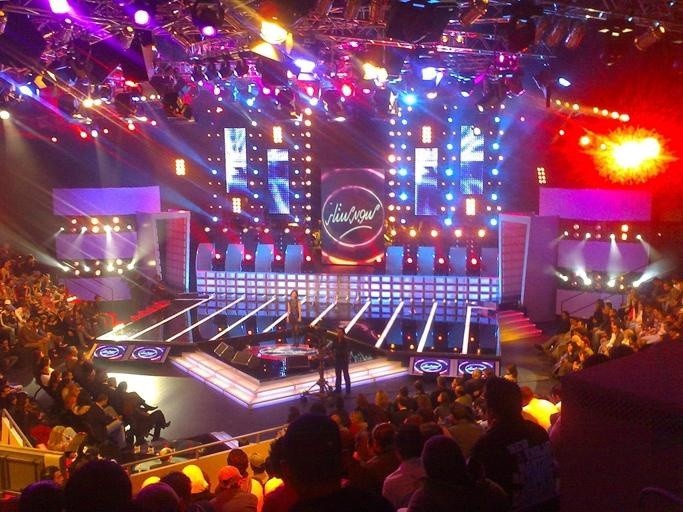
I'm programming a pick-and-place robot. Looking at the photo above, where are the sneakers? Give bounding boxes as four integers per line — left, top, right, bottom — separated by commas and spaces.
534, 343, 543, 350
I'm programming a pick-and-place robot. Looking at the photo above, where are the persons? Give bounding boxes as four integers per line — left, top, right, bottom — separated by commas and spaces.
286, 290, 302, 347
264, 365, 563, 512
537, 276, 682, 380
2, 244, 170, 463
8, 435, 263, 512
330, 328, 351, 399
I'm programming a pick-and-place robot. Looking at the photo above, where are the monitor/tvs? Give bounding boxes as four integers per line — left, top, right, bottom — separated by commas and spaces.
88, 342, 172, 364
408, 355, 500, 377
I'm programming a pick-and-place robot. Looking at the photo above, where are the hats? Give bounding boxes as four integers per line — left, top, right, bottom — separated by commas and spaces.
218, 466, 243, 480
250, 453, 266, 468
182, 465, 209, 493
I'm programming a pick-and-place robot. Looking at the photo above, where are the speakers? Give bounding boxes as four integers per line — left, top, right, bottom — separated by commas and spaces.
213, 342, 256, 370
